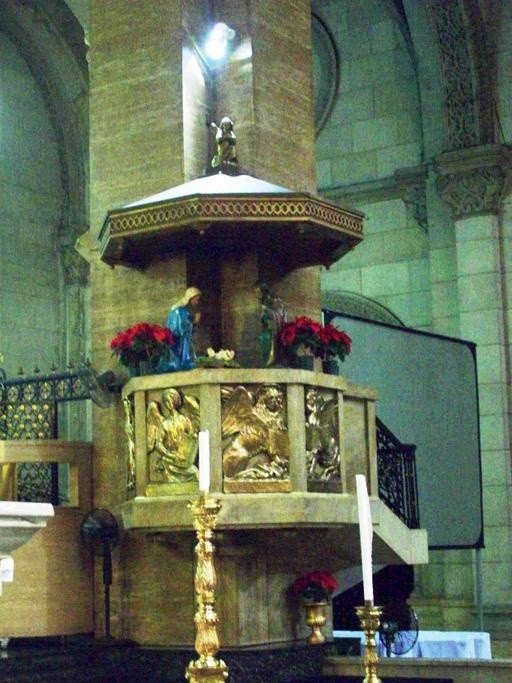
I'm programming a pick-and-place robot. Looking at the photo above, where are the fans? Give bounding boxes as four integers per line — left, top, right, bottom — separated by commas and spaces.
377, 598, 419, 657
80, 503, 138, 648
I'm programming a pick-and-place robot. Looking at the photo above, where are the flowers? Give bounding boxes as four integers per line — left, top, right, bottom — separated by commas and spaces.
110, 321, 178, 366
287, 569, 337, 604
277, 316, 351, 362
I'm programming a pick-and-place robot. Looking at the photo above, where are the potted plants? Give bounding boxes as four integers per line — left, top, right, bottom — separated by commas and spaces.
292, 356, 339, 374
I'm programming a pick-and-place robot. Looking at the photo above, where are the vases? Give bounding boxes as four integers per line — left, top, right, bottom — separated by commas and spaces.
138, 359, 155, 376
305, 602, 329, 645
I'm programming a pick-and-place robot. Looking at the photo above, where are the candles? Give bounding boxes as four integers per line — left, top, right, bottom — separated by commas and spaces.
196, 429, 212, 491
353, 471, 376, 601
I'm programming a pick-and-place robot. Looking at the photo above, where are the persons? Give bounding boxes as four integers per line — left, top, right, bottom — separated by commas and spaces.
211, 117, 238, 167
155, 389, 198, 482
245, 387, 287, 479
258, 283, 288, 368
167, 287, 203, 372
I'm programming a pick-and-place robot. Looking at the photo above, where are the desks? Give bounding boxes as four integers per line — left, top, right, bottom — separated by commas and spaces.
332, 629, 491, 659
